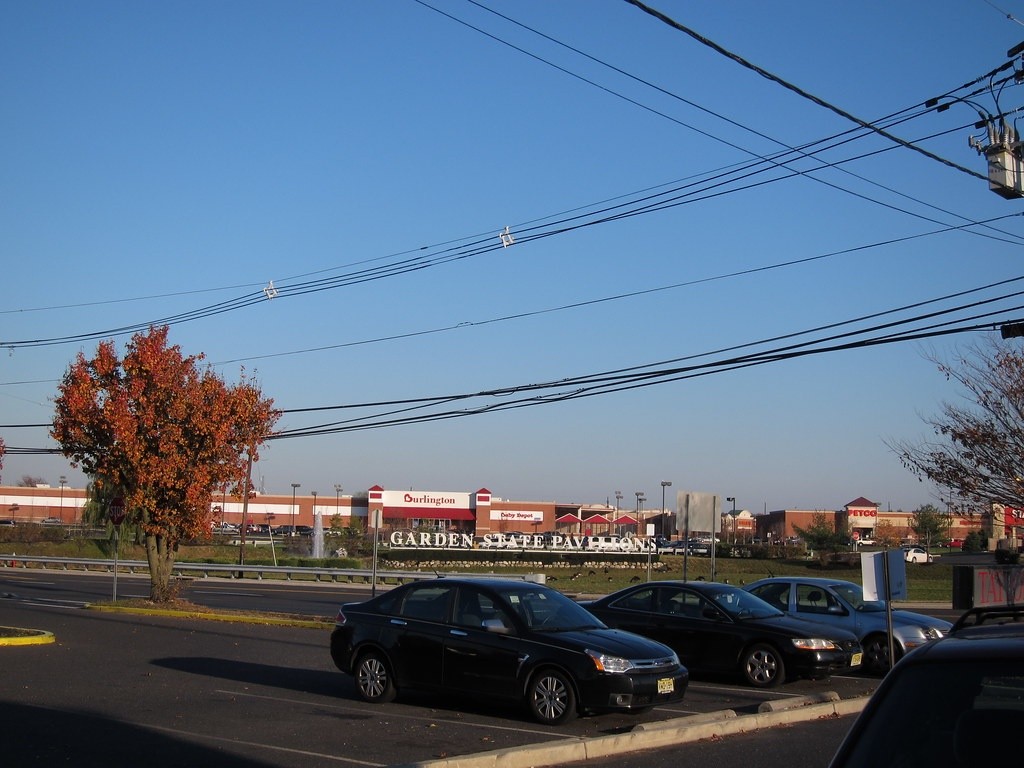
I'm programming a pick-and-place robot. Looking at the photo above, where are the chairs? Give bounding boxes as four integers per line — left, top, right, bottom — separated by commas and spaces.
665, 600, 685, 616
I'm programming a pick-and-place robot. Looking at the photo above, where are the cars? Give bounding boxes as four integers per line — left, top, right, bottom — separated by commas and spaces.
557, 580, 864, 690
501, 530, 811, 557
41, 516, 63, 524
715, 576, 953, 678
0, 519, 18, 529
826, 601, 1023, 768
210, 521, 361, 540
329, 574, 689, 737
899, 541, 934, 565
855, 537, 878, 547
942, 537, 966, 549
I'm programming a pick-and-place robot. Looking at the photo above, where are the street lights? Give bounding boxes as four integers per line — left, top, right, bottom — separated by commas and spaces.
635, 492, 645, 537
949, 491, 955, 537
333, 485, 344, 516
660, 481, 672, 540
638, 498, 648, 533
727, 497, 736, 544
59, 475, 68, 520
312, 491, 319, 530
946, 501, 954, 538
616, 495, 623, 519
291, 483, 302, 532
